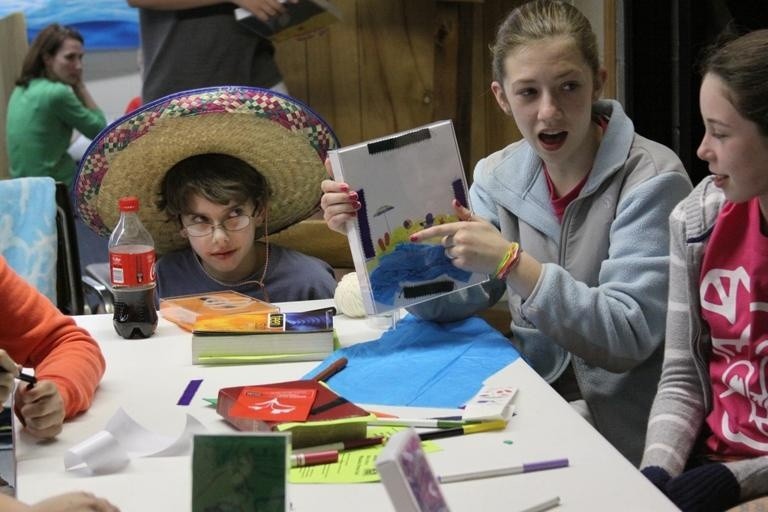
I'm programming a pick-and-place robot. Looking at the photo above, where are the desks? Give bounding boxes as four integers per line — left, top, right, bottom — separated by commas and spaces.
6, 294, 684, 512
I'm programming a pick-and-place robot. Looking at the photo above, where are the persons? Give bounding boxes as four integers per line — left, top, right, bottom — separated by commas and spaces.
6, 22, 108, 197
74, 152, 341, 304
125, 0, 292, 106
0, 255, 106, 441
321, 1, 695, 469
0, 476, 121, 512
639, 29, 768, 512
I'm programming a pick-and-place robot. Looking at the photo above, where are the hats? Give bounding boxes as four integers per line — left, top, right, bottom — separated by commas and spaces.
71, 83, 346, 252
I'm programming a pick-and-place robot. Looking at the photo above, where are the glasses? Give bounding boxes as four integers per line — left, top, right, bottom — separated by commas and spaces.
180, 207, 259, 238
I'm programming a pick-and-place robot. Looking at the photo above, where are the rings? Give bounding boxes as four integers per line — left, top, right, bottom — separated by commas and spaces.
442, 235, 452, 247
468, 210, 473, 220
444, 247, 455, 259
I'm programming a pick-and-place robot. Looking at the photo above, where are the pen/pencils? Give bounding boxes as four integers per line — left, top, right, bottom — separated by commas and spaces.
367, 410, 518, 443
437, 458, 569, 483
521, 496, 561, 512
313, 358, 348, 383
0, 365, 38, 383
290, 435, 386, 467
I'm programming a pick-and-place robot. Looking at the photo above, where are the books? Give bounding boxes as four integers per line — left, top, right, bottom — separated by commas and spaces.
216, 380, 376, 449
232, 1, 342, 47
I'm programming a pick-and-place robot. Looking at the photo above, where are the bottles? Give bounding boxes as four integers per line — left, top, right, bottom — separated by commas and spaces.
106, 197, 159, 342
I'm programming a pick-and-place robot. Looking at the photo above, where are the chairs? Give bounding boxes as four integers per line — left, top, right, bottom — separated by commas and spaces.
0, 177, 86, 313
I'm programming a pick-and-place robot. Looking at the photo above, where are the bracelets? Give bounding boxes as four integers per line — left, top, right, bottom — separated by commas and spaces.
491, 241, 521, 280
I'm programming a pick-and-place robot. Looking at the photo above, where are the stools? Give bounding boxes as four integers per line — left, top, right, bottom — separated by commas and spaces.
80, 260, 125, 316
